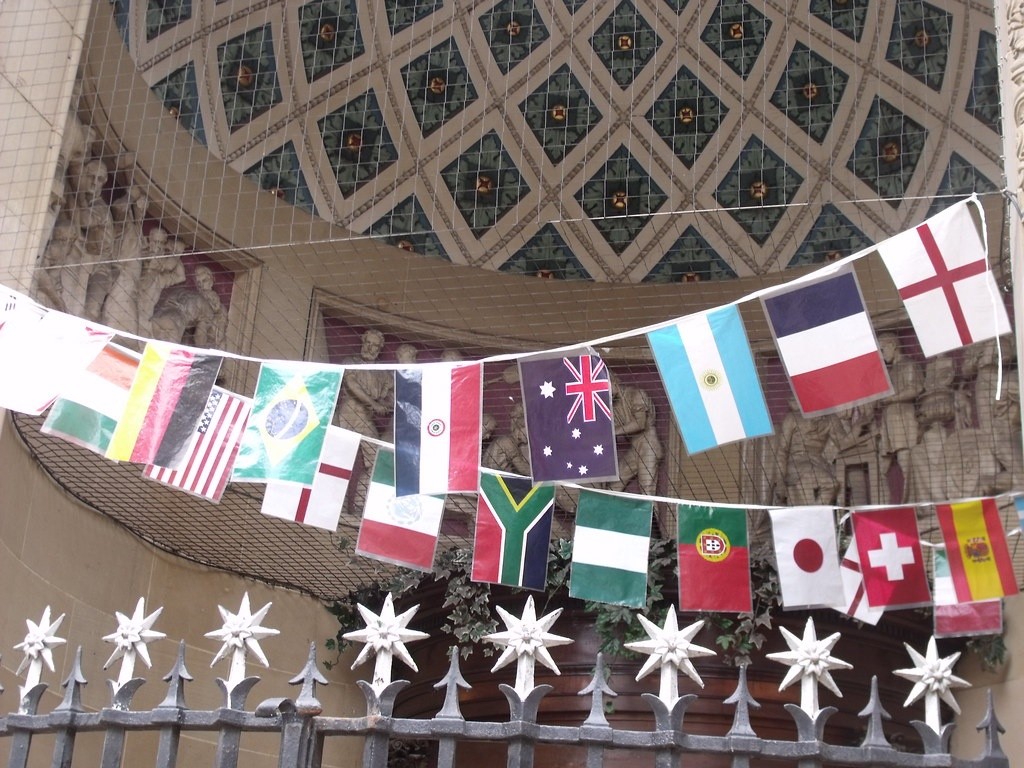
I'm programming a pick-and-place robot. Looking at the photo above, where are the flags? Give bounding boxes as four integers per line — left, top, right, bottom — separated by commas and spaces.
760, 263, 890, 413
396, 364, 480, 497
356, 448, 446, 570
567, 489, 654, 610
519, 353, 617, 483
472, 472, 555, 593
646, 305, 774, 455
830, 500, 1016, 637
235, 363, 342, 486
762, 505, 846, 611
675, 503, 750, 612
102, 341, 223, 472
143, 387, 251, 500
259, 428, 358, 531
877, 205, 1012, 359
46, 344, 139, 453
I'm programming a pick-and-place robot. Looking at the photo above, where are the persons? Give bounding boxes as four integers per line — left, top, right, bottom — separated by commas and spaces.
332, 330, 663, 531
32, 76, 228, 383
778, 332, 1024, 509
